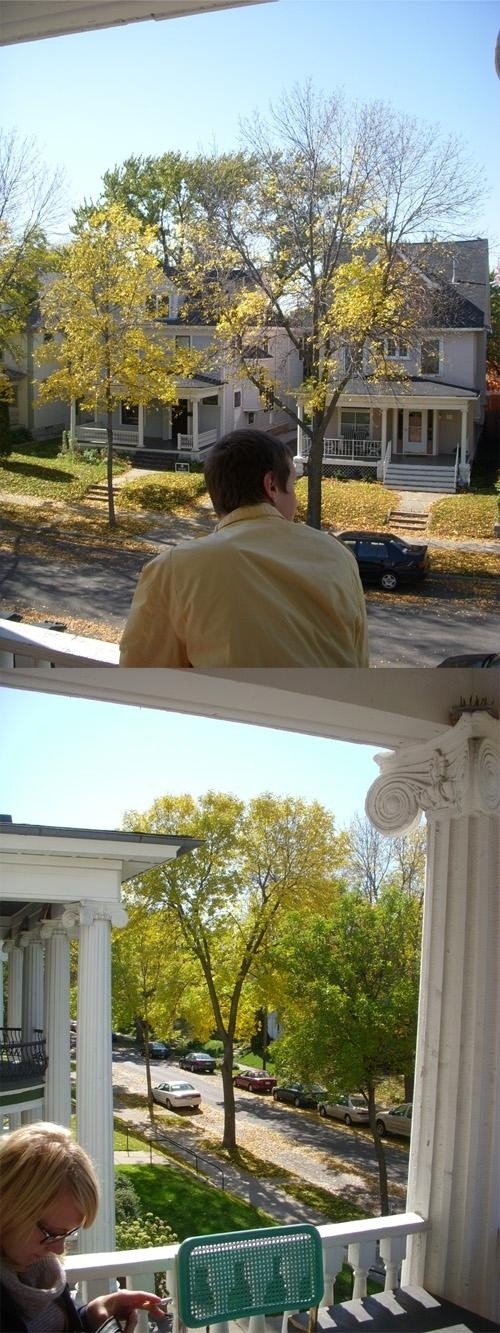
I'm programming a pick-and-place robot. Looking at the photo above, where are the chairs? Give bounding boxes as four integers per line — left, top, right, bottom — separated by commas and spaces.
174, 1223, 324, 1333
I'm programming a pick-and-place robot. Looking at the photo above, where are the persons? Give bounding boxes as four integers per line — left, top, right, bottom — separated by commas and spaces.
0, 1121, 164, 1333
118, 421, 368, 668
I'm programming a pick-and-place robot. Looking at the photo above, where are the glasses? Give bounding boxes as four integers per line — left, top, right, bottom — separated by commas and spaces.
37, 1221, 81, 1246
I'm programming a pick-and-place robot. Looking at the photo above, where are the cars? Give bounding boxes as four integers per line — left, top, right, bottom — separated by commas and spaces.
317, 1095, 382, 1126
178, 1052, 217, 1073
152, 1081, 202, 1109
70, 1021, 76, 1031
272, 1080, 328, 1107
375, 1103, 413, 1138
233, 1069, 277, 1092
140, 1042, 169, 1059
337, 531, 430, 590
